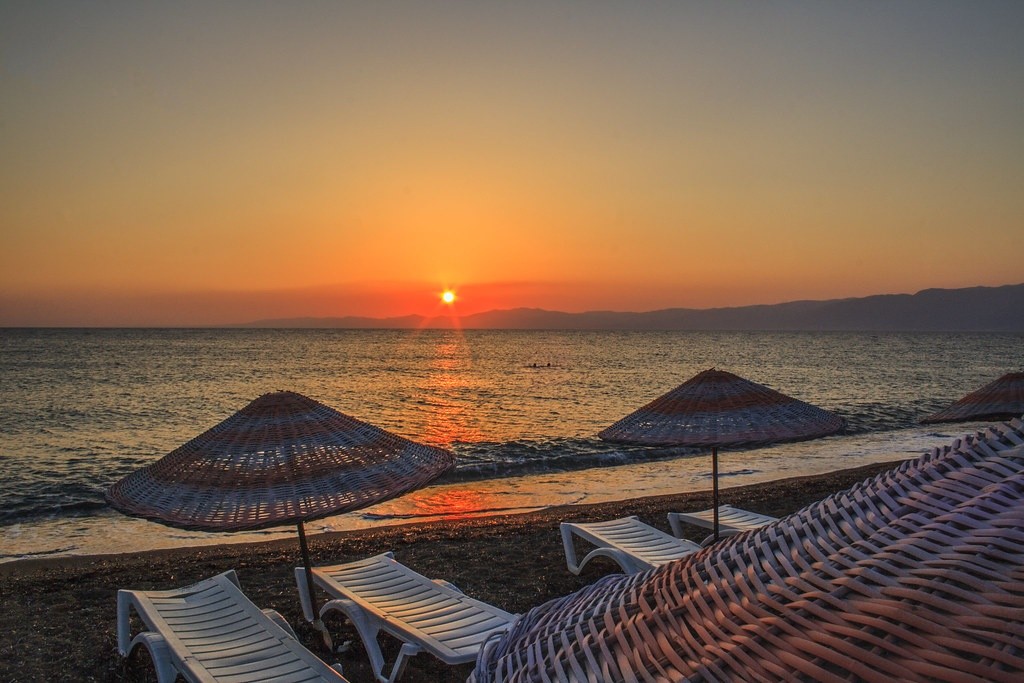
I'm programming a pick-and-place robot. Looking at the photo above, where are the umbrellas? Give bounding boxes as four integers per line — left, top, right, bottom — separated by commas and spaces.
597, 370, 844, 547
919, 371, 1023, 425
466, 412, 1024, 683
106, 390, 454, 620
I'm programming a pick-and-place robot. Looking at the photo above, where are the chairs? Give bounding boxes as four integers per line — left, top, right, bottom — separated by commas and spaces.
117, 568, 351, 683
561, 514, 706, 583
666, 502, 781, 550
307, 549, 526, 683
